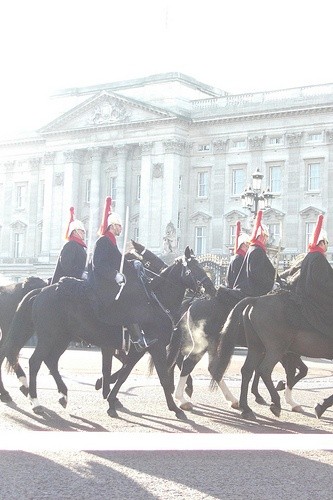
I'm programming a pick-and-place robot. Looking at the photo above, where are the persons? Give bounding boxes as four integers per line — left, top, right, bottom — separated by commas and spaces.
291, 215, 333, 341
81, 198, 158, 349
233, 210, 282, 298
227, 223, 252, 291
51, 206, 88, 286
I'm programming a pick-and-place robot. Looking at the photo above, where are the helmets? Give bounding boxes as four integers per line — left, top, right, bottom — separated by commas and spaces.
234, 220, 250, 252
310, 213, 329, 249
66, 206, 86, 237
99, 195, 123, 236
252, 211, 270, 242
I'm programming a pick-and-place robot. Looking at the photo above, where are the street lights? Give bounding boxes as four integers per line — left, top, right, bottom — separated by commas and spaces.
240, 166, 276, 223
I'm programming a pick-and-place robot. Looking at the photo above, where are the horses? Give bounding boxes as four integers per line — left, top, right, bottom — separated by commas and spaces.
0, 239, 333, 420
